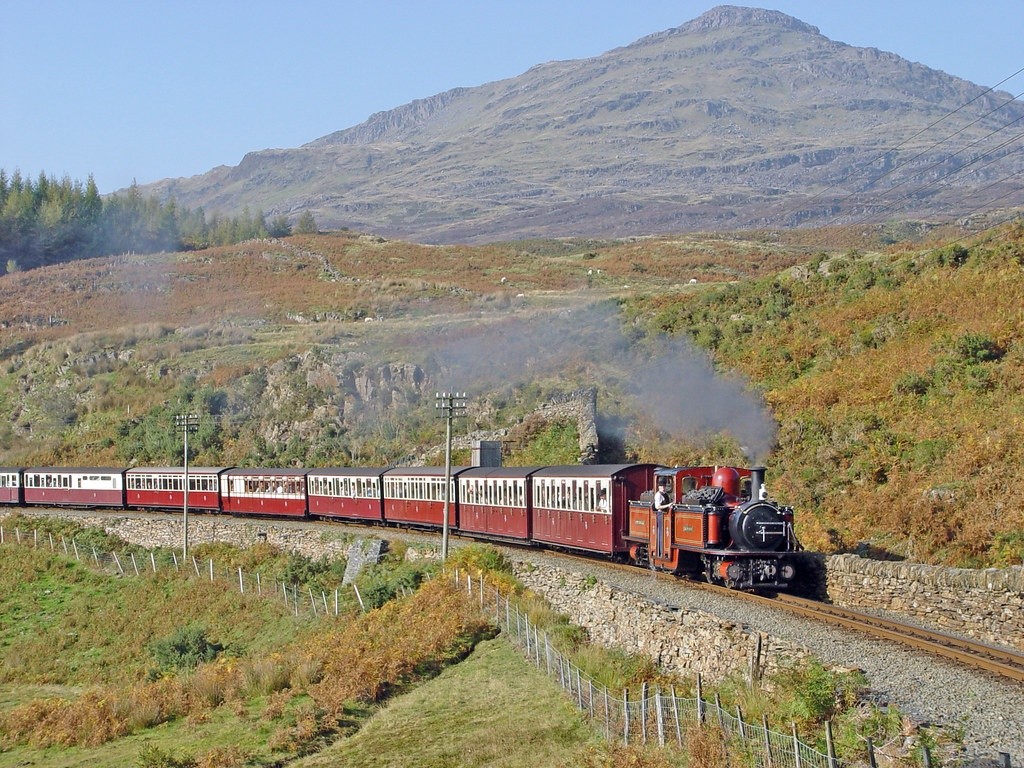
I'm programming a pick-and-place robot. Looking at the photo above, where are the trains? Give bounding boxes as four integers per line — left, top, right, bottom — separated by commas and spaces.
1, 459, 807, 596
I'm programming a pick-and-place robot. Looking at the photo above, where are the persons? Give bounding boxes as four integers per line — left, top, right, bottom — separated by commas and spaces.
34, 476, 66, 487
740, 480, 752, 499
313, 483, 377, 497
542, 488, 608, 512
386, 485, 446, 499
130, 479, 217, 491
249, 482, 304, 494
654, 481, 673, 556
468, 489, 523, 507
